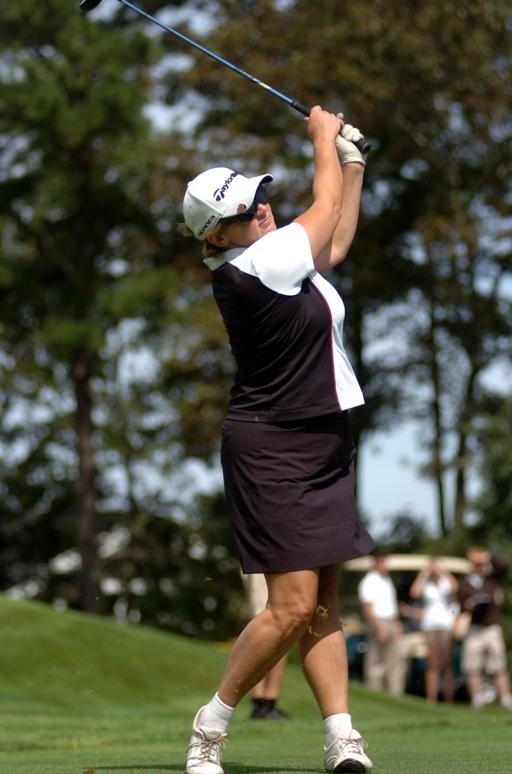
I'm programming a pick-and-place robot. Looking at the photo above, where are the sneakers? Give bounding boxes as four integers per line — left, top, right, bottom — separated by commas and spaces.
184, 706, 228, 774
323, 730, 373, 774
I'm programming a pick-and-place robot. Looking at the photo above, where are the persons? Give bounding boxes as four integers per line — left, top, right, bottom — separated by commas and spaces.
411, 554, 460, 705
180, 104, 374, 774
358, 545, 407, 697
237, 561, 294, 720
457, 538, 511, 708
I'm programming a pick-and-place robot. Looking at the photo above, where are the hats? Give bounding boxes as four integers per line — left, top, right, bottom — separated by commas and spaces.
182, 165, 273, 242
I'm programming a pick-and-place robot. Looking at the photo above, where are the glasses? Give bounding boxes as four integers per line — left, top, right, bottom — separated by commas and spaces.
215, 185, 269, 238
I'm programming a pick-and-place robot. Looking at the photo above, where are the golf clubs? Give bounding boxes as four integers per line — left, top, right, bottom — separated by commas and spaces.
80, 0, 372, 155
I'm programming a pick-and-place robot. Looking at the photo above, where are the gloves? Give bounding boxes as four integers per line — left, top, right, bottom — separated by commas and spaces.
335, 124, 370, 168
